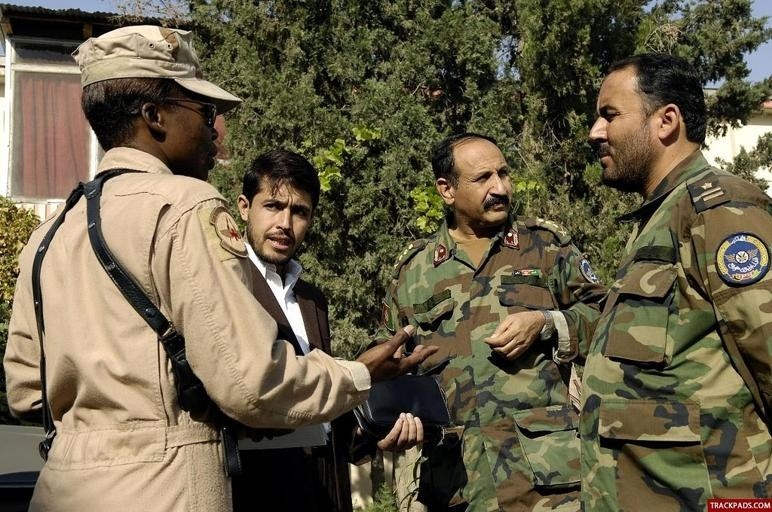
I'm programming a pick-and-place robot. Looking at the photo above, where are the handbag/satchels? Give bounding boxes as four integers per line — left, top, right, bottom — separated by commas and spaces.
346, 373, 450, 440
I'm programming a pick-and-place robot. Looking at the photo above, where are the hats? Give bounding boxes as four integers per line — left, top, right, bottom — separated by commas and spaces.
71, 25, 243, 116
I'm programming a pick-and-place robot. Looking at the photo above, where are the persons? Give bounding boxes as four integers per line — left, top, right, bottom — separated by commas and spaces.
1, 23, 771, 511
